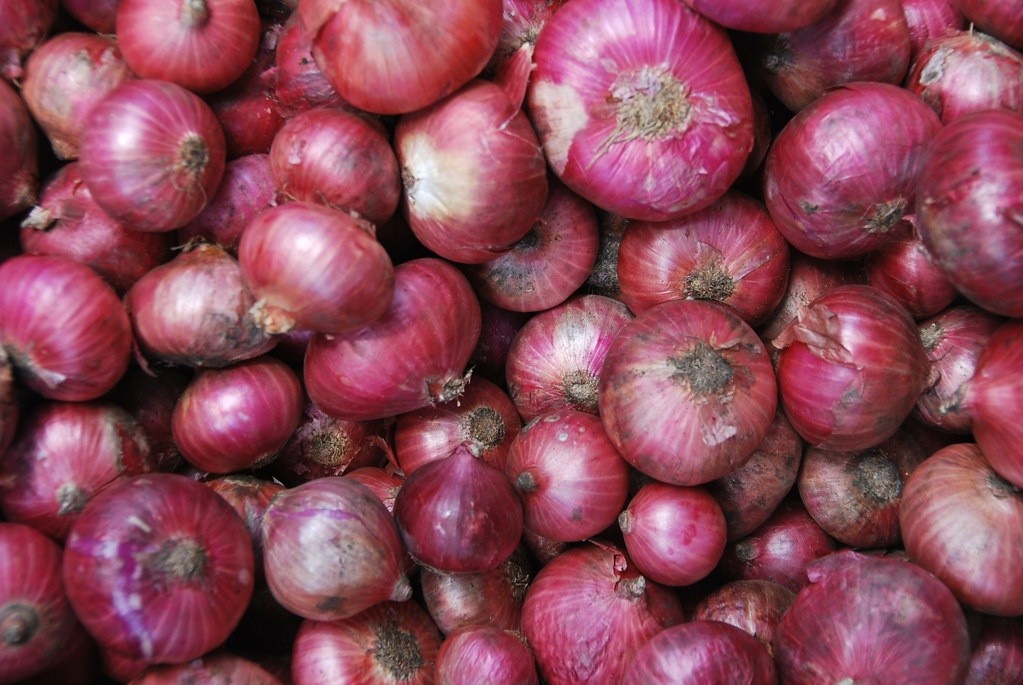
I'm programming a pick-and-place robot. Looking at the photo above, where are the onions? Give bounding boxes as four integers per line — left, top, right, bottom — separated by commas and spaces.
0, 0, 1023, 685
